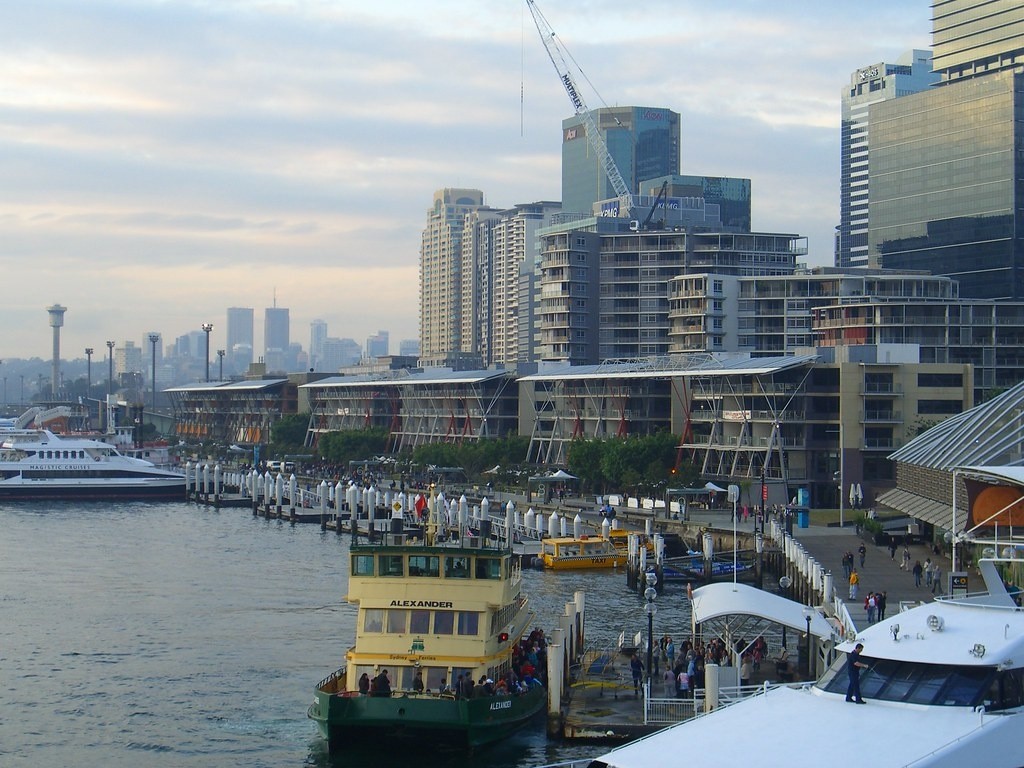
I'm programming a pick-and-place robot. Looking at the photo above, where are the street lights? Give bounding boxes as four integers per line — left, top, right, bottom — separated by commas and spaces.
801, 605, 815, 681
944, 532, 975, 572
38, 373, 43, 392
779, 575, 794, 648
20, 375, 25, 404
3, 377, 8, 403
107, 341, 115, 395
644, 573, 657, 699
202, 323, 213, 382
218, 350, 226, 382
149, 335, 159, 413
982, 547, 1017, 582
85, 348, 93, 396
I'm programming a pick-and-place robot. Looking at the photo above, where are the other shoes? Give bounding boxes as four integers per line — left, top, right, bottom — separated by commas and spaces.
856, 699, 866, 704
845, 698, 856, 702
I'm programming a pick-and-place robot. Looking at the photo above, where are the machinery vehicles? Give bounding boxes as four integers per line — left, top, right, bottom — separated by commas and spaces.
521, 1, 669, 233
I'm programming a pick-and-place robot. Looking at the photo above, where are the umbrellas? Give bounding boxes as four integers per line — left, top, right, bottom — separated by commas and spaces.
856, 481, 863, 510
704, 481, 728, 503
849, 482, 857, 510
363, 454, 433, 476
484, 464, 575, 488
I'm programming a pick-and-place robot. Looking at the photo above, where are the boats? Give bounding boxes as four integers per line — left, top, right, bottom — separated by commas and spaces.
0, 401, 191, 502
539, 466, 1024, 768
645, 558, 756, 582
530, 528, 652, 570
307, 508, 548, 756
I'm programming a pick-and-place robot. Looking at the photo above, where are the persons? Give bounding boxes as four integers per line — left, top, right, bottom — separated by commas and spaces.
864, 591, 887, 623
888, 536, 897, 561
845, 643, 868, 704
900, 546, 910, 572
776, 649, 793, 682
455, 561, 465, 572
450, 479, 517, 517
848, 567, 861, 600
359, 669, 392, 698
905, 533, 912, 550
842, 551, 854, 581
923, 558, 933, 588
864, 506, 879, 520
600, 503, 616, 523
412, 670, 425, 694
239, 455, 433, 497
912, 561, 922, 588
930, 564, 944, 598
858, 543, 867, 568
548, 482, 573, 503
731, 500, 784, 528
630, 632, 765, 699
790, 495, 796, 506
439, 627, 548, 699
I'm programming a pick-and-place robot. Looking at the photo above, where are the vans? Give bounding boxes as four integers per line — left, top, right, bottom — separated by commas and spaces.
260, 461, 297, 474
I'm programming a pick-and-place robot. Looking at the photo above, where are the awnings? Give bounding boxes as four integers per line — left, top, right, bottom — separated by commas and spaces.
875, 487, 970, 535
